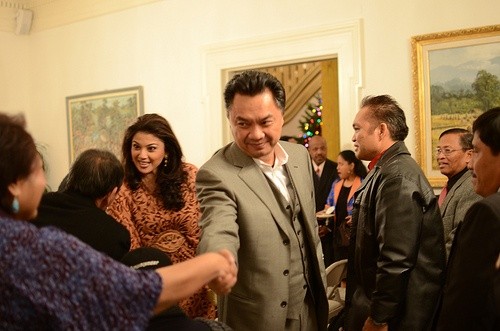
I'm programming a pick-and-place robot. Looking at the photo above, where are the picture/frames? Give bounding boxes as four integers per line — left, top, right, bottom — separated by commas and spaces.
411, 25, 500, 187
66, 86, 144, 171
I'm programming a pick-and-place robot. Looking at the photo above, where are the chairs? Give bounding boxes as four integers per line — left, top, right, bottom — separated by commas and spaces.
321, 259, 348, 319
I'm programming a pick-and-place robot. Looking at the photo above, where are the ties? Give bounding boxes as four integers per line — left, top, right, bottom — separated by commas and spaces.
438, 182, 448, 208
316, 167, 319, 177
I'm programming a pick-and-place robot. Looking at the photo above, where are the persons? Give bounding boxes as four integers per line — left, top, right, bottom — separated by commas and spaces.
435, 127, 484, 264
280, 136, 298, 143
341, 94, 446, 331
308, 135, 339, 242
424, 107, 500, 331
26, 148, 131, 262
316, 149, 368, 263
0, 110, 239, 331
104, 114, 216, 322
193, 70, 330, 330
120, 246, 233, 331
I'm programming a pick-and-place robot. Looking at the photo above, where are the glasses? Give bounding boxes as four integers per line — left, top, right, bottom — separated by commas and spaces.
435, 147, 470, 156
